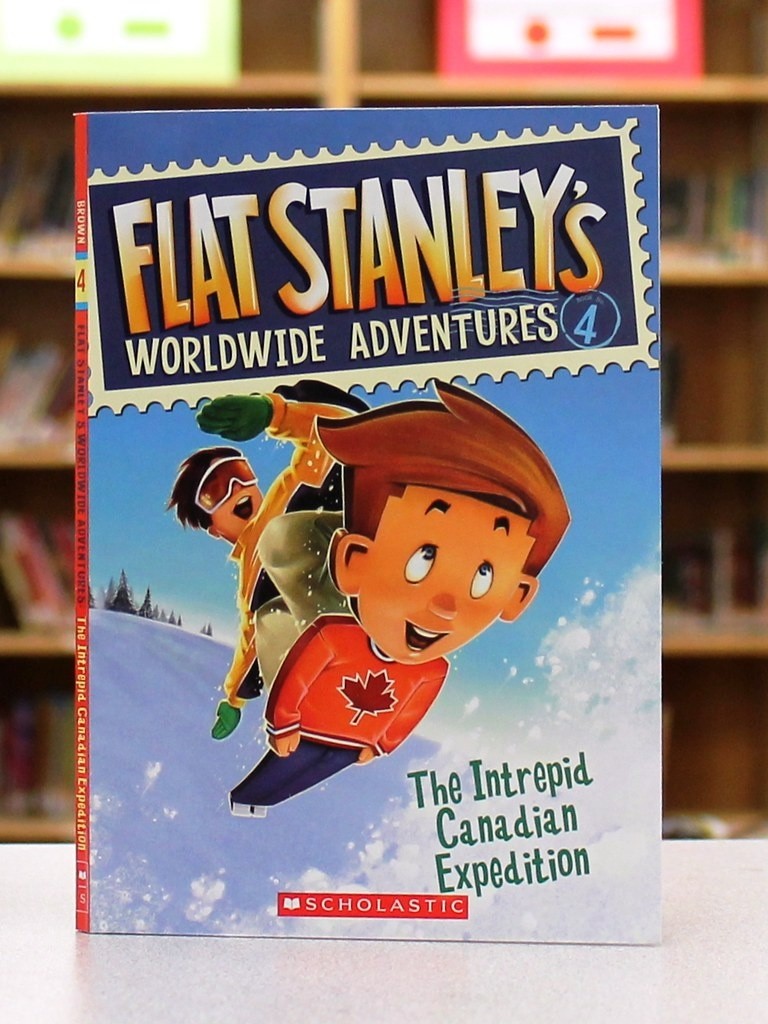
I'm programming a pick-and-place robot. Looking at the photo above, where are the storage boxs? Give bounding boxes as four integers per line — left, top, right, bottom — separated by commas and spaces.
434, 0, 705, 79
0, 0, 241, 83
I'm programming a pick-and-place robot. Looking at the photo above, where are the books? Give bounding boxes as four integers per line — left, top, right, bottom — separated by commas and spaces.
71, 104, 663, 945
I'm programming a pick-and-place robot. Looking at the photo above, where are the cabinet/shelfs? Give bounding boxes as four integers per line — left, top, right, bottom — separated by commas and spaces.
0, 0, 768, 847
